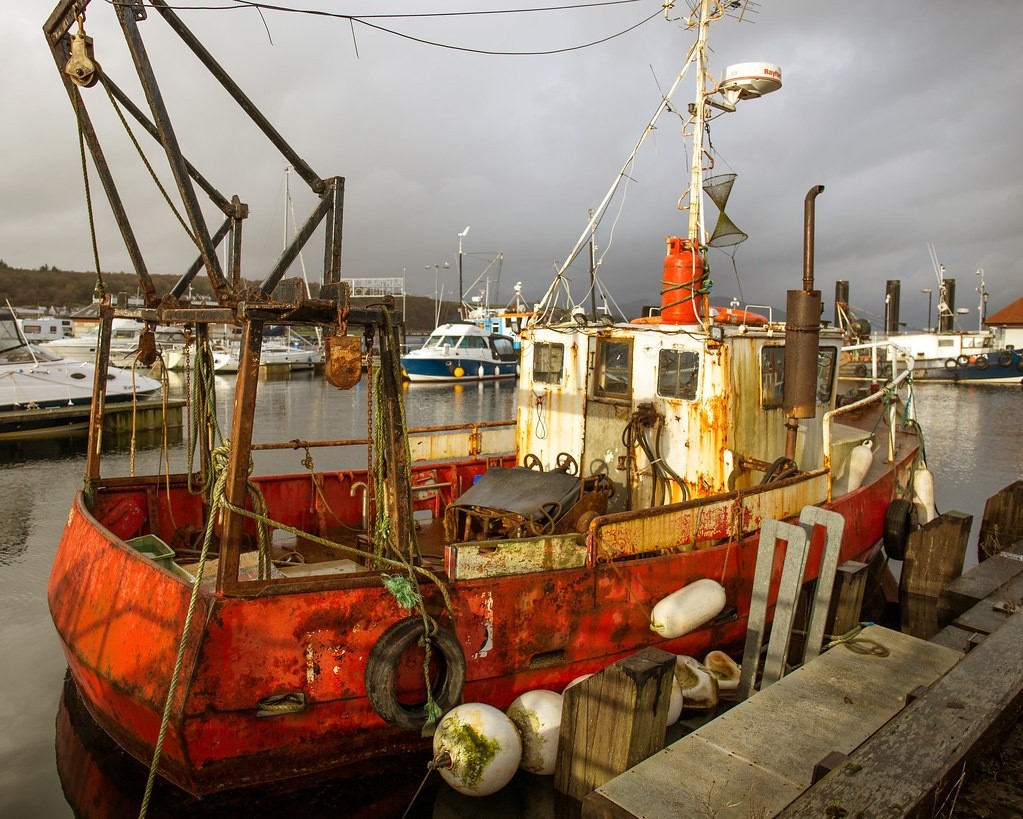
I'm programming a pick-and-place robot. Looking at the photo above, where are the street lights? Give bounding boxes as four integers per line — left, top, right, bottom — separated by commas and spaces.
425, 265, 450, 328
975, 268, 983, 330
921, 289, 931, 334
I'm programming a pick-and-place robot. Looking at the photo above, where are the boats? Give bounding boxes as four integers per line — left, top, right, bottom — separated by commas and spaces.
0, 167, 382, 411
398, 225, 550, 383
839, 243, 1022, 385
42, 0, 932, 806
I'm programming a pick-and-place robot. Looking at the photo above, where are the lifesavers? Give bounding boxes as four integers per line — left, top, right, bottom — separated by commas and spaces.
854, 363, 892, 379
882, 497, 919, 561
363, 614, 467, 733
629, 304, 768, 327
944, 353, 1023, 372
